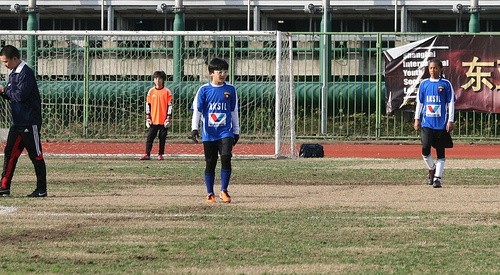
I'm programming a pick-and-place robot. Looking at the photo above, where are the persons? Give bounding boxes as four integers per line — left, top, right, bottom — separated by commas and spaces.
190, 58, 240, 203
140, 70, 173, 160
414, 58, 455, 188
0, 45, 48, 197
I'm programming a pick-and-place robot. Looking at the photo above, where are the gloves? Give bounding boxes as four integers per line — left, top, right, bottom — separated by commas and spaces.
163, 116, 172, 129
192, 130, 199, 142
145, 115, 152, 129
231, 135, 239, 145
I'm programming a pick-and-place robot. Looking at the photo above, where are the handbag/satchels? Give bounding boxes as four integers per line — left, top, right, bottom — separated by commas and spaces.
298, 143, 324, 158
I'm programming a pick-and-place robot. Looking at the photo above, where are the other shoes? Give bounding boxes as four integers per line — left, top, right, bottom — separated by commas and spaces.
218, 191, 231, 203
140, 154, 150, 160
427, 163, 436, 185
157, 152, 163, 161
206, 195, 216, 203
432, 176, 442, 188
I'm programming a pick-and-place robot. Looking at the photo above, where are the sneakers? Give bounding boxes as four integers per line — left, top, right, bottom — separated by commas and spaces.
0, 187, 10, 197
26, 188, 47, 197
